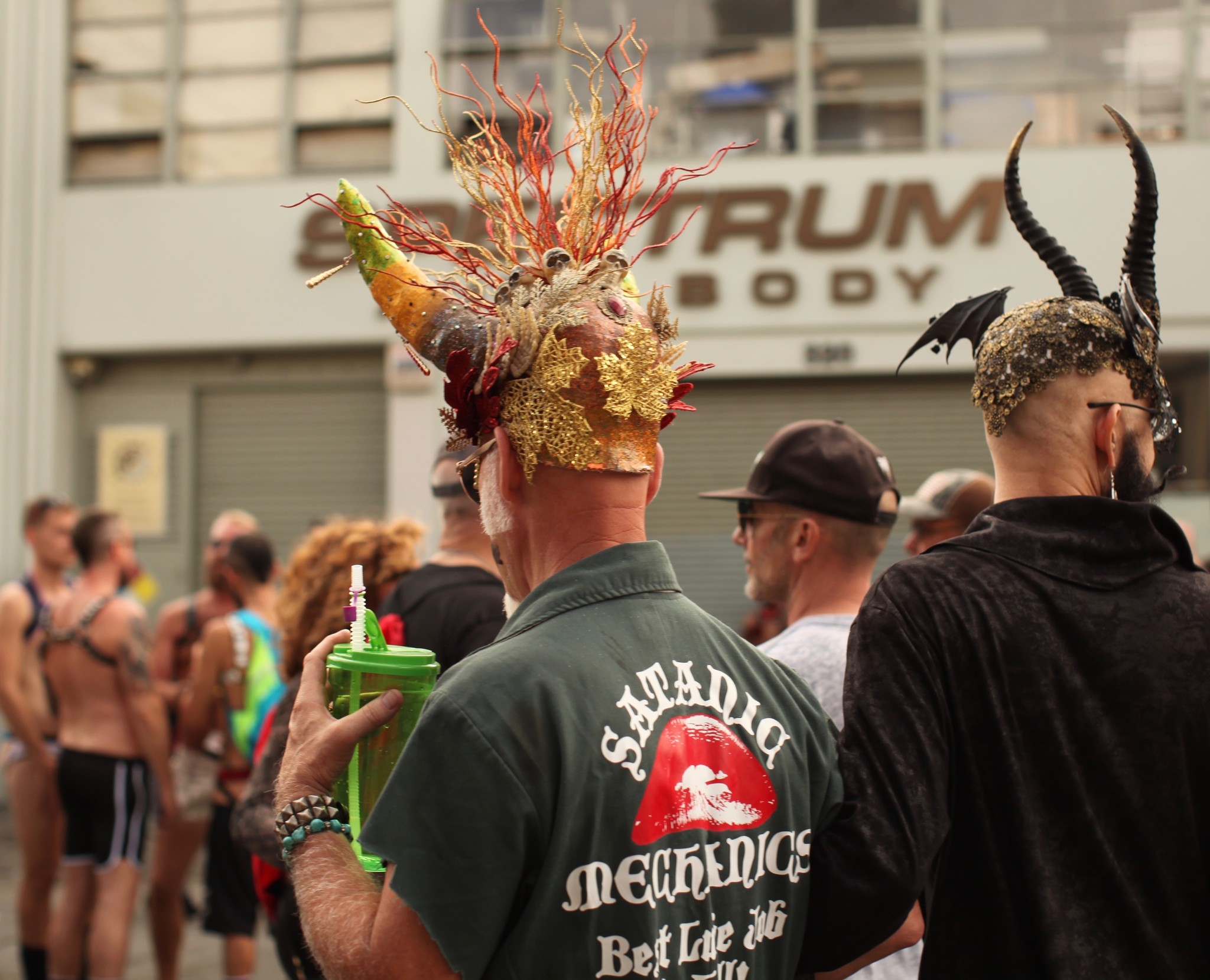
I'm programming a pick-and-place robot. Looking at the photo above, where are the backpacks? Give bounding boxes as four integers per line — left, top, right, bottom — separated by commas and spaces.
231, 576, 495, 867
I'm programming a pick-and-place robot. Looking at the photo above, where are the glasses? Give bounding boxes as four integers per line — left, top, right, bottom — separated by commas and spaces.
911, 523, 967, 540
737, 502, 806, 532
1088, 402, 1178, 441
456, 438, 495, 505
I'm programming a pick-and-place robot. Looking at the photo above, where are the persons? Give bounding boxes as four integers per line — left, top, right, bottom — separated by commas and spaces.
1, 265, 1209, 980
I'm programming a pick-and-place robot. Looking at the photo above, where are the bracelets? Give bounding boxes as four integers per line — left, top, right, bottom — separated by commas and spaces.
281, 819, 354, 868
273, 794, 350, 849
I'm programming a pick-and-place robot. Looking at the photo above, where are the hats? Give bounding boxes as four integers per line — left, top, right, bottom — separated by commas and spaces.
895, 103, 1171, 438
429, 444, 477, 496
281, 7, 757, 474
900, 468, 995, 519
698, 419, 901, 526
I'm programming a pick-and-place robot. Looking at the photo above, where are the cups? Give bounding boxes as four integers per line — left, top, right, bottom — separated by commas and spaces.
325, 564, 441, 874
